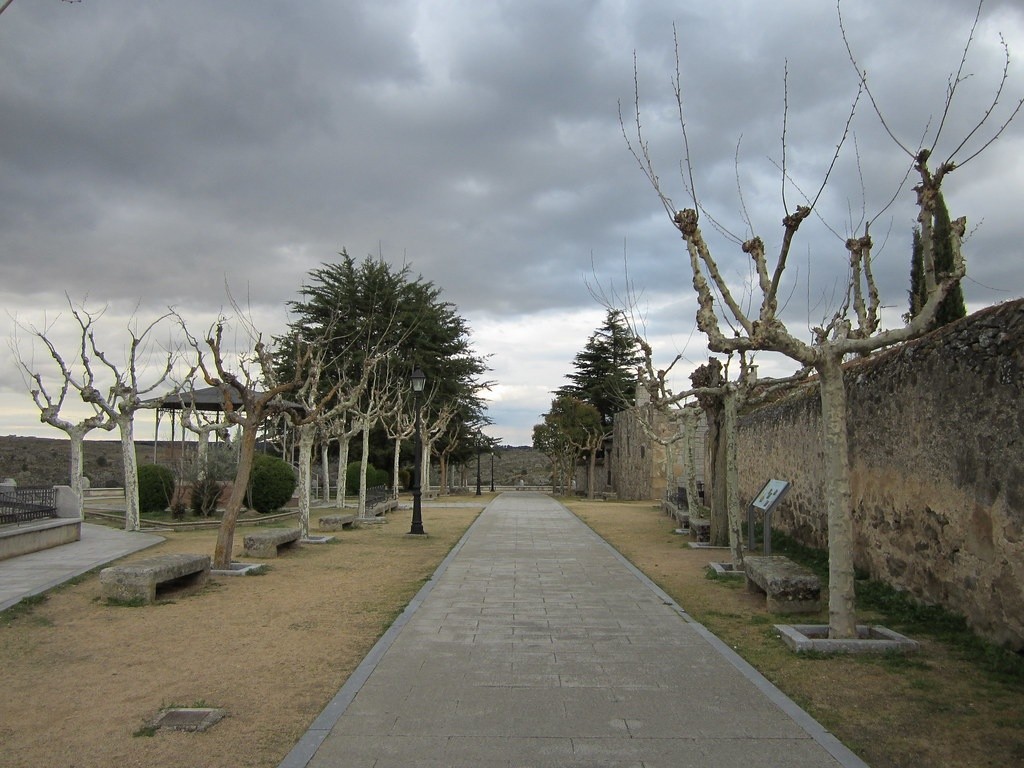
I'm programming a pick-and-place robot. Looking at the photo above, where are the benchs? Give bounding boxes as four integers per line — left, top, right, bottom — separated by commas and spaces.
664, 485, 711, 541
741, 554, 823, 615
318, 514, 354, 531
241, 526, 303, 557
365, 485, 399, 516
99, 554, 210, 601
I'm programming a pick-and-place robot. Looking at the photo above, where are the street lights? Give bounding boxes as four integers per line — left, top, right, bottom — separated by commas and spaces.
476, 427, 482, 496
490, 450, 496, 491
408, 364, 429, 535
